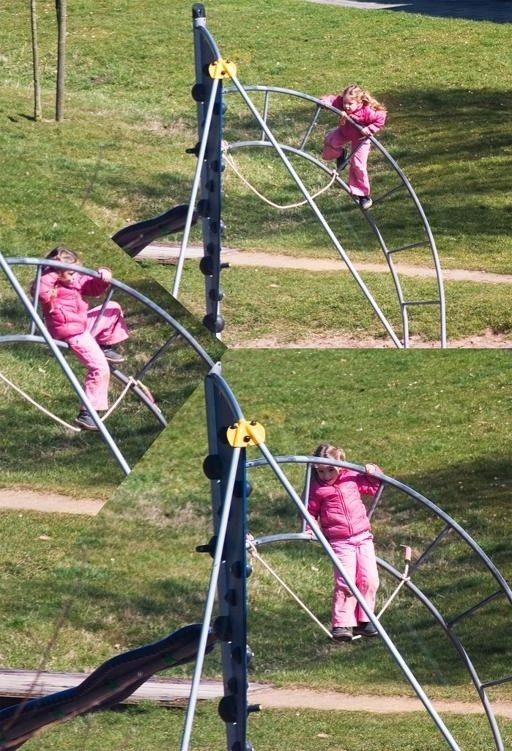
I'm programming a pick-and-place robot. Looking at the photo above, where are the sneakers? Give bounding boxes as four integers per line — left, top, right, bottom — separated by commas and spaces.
102, 349, 124, 364
352, 621, 379, 638
331, 625, 353, 641
358, 194, 373, 211
75, 412, 100, 432
337, 148, 348, 170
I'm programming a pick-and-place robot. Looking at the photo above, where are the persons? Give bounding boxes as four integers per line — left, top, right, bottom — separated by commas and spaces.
30, 247, 132, 435
314, 82, 389, 214
298, 443, 385, 642
